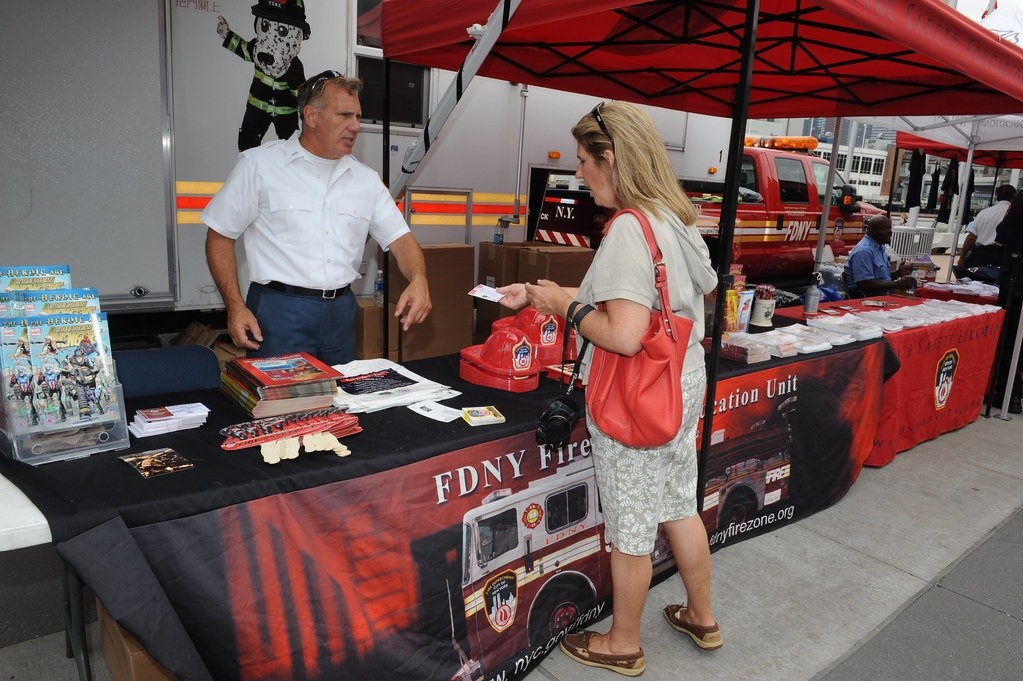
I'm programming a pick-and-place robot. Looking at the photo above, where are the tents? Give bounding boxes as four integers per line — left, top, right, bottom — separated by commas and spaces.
887, 114, 1023, 230
366, 0, 1023, 361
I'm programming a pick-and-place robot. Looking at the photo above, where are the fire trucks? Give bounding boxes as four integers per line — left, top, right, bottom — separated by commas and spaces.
443, 394, 798, 681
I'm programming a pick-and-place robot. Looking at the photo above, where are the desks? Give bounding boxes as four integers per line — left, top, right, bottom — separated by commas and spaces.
0, 280, 1006, 681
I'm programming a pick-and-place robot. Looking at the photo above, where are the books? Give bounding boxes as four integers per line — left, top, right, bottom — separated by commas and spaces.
219, 352, 344, 421
701, 299, 985, 367
463, 406, 505, 425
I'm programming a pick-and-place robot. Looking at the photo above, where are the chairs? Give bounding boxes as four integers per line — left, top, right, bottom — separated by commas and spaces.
842, 272, 850, 300
114, 342, 221, 399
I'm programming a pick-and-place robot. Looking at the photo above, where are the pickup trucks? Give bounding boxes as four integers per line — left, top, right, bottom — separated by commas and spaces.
534, 137, 893, 283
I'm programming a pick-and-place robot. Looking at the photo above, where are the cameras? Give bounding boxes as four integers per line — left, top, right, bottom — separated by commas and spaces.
534, 395, 580, 450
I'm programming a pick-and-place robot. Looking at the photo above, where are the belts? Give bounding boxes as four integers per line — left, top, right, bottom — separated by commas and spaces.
265, 282, 352, 300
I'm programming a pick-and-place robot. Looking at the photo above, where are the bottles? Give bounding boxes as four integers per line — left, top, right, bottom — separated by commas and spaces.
493, 218, 504, 245
802, 282, 820, 317
372, 270, 383, 304
905, 268, 918, 294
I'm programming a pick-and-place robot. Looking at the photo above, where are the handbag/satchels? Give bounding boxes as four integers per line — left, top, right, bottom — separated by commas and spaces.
585, 209, 694, 448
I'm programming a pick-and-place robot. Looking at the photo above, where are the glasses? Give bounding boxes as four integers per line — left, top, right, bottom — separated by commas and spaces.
299, 71, 343, 117
589, 101, 614, 152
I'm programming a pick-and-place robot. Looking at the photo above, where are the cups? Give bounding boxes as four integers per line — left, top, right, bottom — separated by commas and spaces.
749, 299, 775, 327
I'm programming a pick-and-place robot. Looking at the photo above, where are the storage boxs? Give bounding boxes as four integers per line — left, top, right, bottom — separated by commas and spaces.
96, 593, 175, 681
358, 236, 595, 362
178, 319, 247, 377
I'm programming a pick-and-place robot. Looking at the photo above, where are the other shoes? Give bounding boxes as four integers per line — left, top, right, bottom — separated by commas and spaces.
663, 603, 723, 650
984, 394, 1021, 413
559, 628, 645, 676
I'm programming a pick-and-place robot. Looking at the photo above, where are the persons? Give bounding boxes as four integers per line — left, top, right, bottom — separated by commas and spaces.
991, 188, 1023, 412
958, 185, 1016, 264
846, 214, 917, 298
496, 102, 724, 677
200, 71, 433, 365
